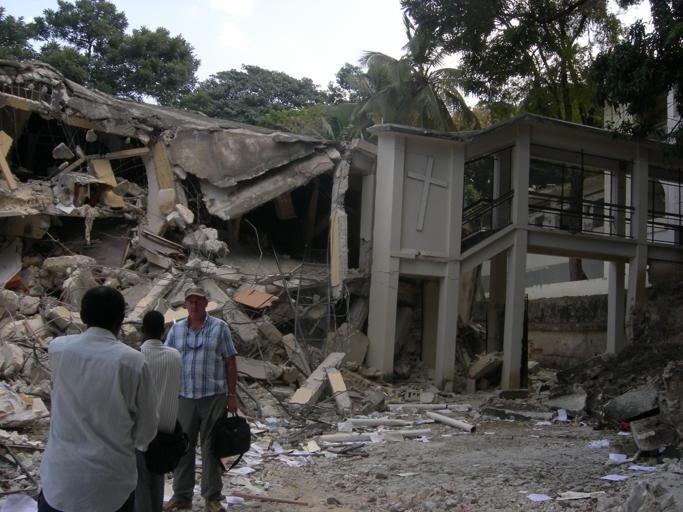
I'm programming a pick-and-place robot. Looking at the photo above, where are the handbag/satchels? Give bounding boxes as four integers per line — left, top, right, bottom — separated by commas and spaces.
211, 411, 252, 473
145, 421, 191, 475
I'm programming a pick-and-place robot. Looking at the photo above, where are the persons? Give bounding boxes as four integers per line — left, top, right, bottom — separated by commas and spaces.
134, 310, 184, 512
165, 286, 240, 512
36, 286, 156, 512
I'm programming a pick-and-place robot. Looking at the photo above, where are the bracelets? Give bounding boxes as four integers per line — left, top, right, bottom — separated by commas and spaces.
228, 393, 237, 398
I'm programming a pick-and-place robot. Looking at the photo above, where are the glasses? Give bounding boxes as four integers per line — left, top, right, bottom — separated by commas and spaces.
186, 340, 203, 350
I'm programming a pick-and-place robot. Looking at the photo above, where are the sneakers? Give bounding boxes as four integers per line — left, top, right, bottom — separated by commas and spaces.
163, 495, 193, 511
206, 499, 227, 512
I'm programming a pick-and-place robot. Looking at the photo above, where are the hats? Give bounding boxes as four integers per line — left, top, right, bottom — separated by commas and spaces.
185, 286, 207, 299
